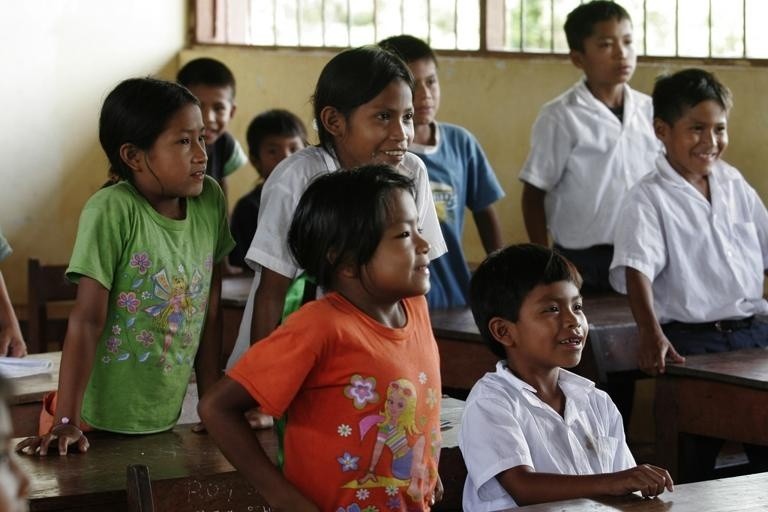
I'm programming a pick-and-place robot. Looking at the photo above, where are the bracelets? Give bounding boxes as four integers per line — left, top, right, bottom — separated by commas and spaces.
61, 417, 70, 424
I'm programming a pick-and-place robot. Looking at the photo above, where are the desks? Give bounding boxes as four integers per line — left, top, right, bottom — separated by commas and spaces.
655, 339, 767, 481
219, 272, 259, 362
1, 351, 65, 443
496, 470, 768, 512
8, 390, 468, 511
426, 291, 644, 411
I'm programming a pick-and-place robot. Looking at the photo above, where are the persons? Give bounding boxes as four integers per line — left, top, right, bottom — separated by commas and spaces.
197, 164, 444, 512
457, 243, 676, 512
176, 58, 249, 277
608, 69, 768, 485
0, 232, 27, 359
378, 34, 506, 309
15, 78, 236, 456
229, 108, 313, 272
519, 0, 667, 435
225, 45, 449, 431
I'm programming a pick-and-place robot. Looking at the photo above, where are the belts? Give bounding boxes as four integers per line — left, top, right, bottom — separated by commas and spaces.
661, 314, 755, 336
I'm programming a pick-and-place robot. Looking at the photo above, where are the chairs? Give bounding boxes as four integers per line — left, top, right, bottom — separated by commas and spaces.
586, 316, 753, 480
122, 456, 284, 512
20, 258, 81, 356
